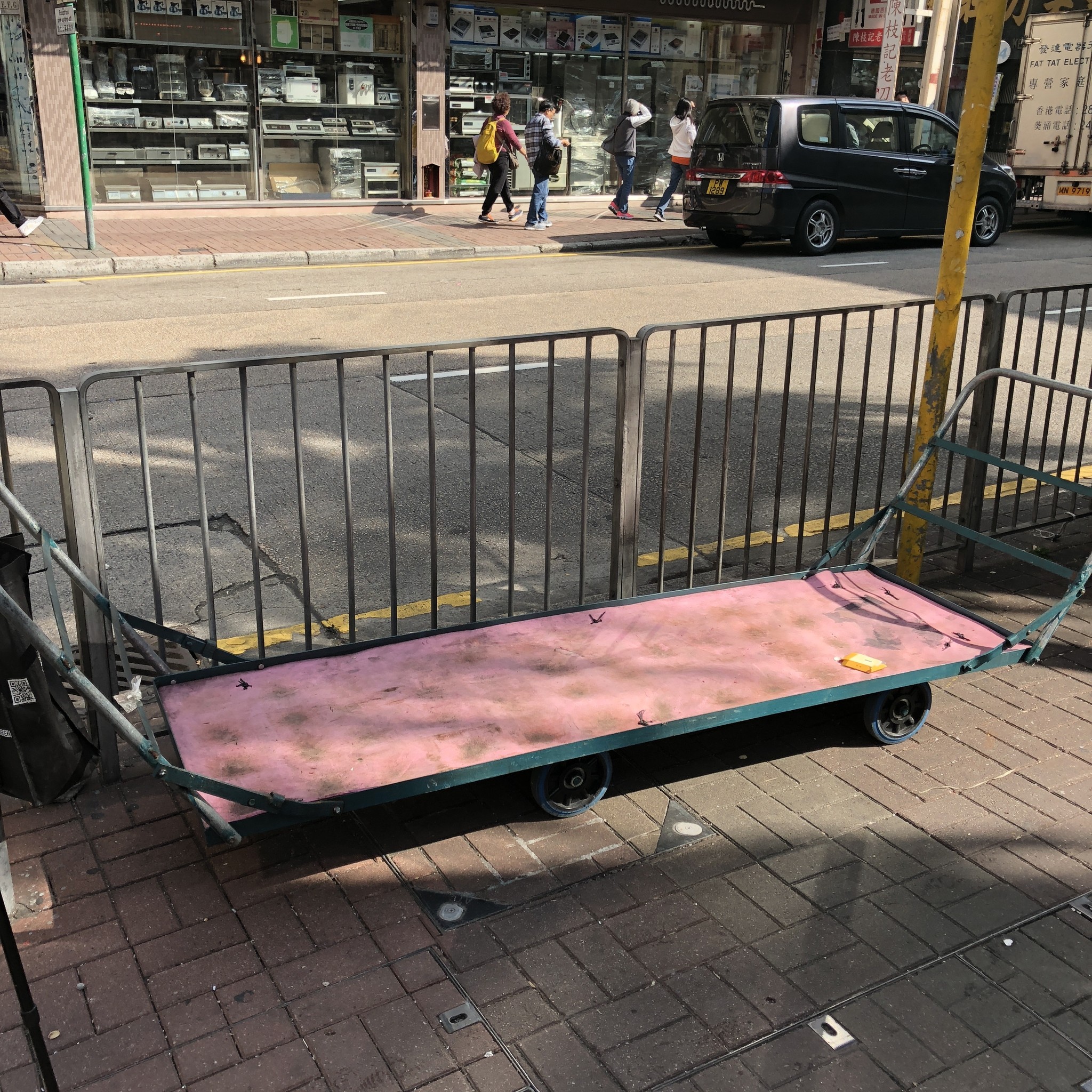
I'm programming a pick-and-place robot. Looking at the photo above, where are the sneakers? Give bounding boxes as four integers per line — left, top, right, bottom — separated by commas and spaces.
508, 208, 523, 221
538, 219, 552, 226
653, 211, 666, 222
608, 201, 619, 216
616, 210, 633, 219
478, 213, 499, 224
524, 222, 546, 230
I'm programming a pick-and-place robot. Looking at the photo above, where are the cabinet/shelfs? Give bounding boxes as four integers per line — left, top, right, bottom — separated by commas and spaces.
79, 36, 251, 164
256, 45, 403, 140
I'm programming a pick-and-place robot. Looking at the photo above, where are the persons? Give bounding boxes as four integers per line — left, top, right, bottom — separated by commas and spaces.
895, 91, 916, 145
846, 122, 859, 148
654, 97, 697, 222
524, 101, 570, 231
478, 92, 528, 223
608, 99, 652, 219
0, 182, 44, 237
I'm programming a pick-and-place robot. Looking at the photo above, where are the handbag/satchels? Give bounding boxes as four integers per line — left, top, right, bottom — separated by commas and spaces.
600, 132, 615, 155
533, 117, 562, 175
508, 152, 519, 169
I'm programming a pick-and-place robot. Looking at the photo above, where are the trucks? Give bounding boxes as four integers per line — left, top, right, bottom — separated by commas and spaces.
1004, 8, 1092, 219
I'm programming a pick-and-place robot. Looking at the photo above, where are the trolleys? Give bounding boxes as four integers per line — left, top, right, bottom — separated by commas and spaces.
0, 368, 1092, 855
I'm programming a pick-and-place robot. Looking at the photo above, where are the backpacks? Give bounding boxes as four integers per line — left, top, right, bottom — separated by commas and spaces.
476, 116, 506, 164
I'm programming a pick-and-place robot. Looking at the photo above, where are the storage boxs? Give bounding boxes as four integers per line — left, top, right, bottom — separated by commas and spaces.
450, 3, 622, 51
256, 0, 400, 55
269, 163, 331, 200
628, 16, 686, 55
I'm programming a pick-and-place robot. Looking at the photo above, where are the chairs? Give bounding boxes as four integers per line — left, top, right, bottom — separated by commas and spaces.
864, 121, 893, 151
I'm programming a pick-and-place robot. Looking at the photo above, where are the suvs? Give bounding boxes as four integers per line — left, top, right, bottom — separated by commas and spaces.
683, 94, 1019, 258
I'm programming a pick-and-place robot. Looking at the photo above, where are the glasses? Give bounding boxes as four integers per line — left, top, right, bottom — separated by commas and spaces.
548, 109, 555, 116
